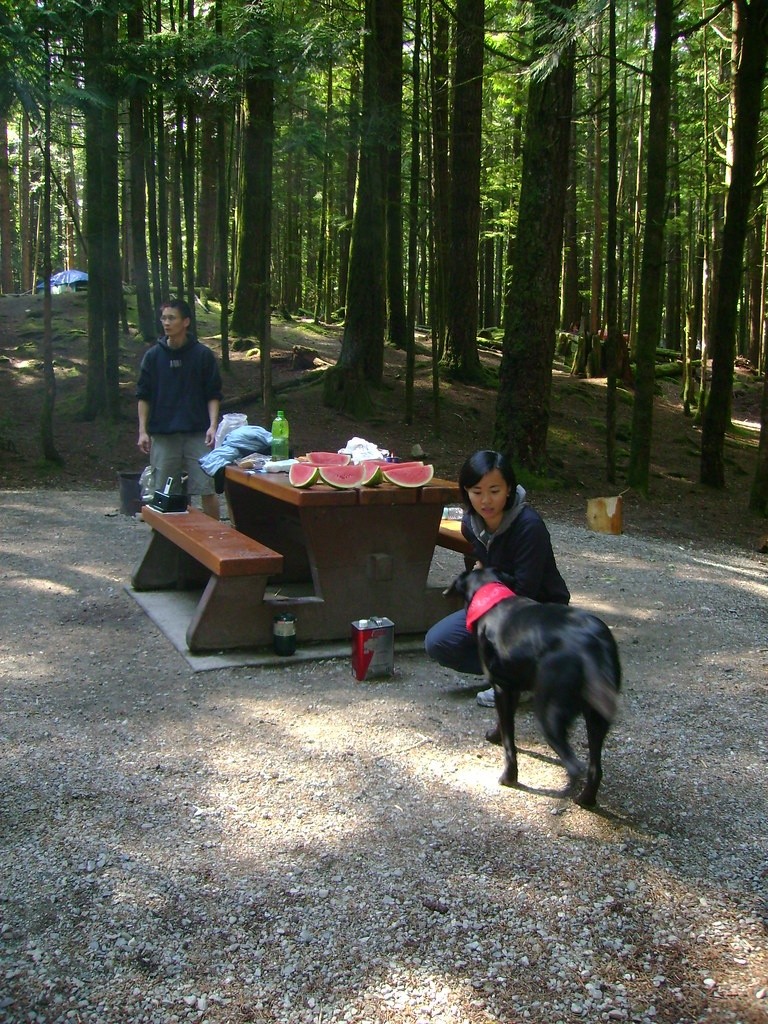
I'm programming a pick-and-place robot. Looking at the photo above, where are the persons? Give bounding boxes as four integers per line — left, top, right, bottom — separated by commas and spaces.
137, 298, 222, 523
427, 449, 571, 706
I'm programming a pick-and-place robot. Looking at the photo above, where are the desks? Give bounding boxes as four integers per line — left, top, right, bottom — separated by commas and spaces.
132, 463, 478, 649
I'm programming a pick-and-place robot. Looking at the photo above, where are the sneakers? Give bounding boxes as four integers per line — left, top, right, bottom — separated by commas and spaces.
477, 687, 532, 707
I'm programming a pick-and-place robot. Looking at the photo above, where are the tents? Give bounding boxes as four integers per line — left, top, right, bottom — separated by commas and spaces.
36, 269, 88, 288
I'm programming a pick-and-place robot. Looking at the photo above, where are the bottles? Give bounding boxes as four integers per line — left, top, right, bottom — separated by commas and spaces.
273, 604, 297, 656
271, 411, 289, 461
441, 508, 463, 519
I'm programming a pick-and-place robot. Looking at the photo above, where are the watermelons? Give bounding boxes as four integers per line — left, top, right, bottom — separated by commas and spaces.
289, 452, 434, 490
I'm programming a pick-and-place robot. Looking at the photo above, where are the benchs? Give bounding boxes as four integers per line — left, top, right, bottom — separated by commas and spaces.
142, 504, 284, 576
436, 519, 478, 556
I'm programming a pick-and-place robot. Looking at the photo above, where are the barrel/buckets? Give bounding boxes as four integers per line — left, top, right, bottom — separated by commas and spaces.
119, 473, 145, 517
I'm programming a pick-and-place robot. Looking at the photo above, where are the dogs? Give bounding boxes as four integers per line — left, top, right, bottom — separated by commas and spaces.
441, 564, 623, 807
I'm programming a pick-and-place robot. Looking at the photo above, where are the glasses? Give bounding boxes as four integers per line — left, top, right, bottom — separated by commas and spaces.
160, 316, 186, 321
